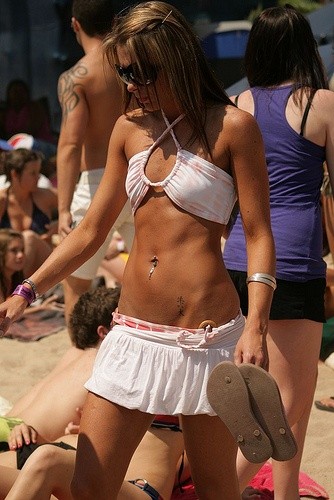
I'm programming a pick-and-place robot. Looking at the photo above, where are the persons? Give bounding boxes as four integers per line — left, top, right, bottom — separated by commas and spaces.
221, 3, 333, 500
0, 149, 59, 245
222, 166, 334, 365
57, 0, 135, 322
0, 78, 60, 191
0, 412, 185, 500
314, 395, 334, 411
0, 228, 57, 321
0, 0, 277, 500
0, 286, 123, 451
100, 229, 132, 286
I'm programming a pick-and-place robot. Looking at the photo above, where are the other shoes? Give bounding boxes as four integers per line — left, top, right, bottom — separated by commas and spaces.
206, 360, 273, 463
315, 396, 334, 412
236, 364, 298, 461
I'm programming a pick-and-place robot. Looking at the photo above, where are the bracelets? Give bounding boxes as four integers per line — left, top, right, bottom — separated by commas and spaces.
117, 239, 125, 253
245, 272, 276, 284
245, 277, 276, 291
10, 284, 34, 307
20, 279, 39, 302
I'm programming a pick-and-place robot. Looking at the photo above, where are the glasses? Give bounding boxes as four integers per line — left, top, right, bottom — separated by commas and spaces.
114, 62, 159, 88
